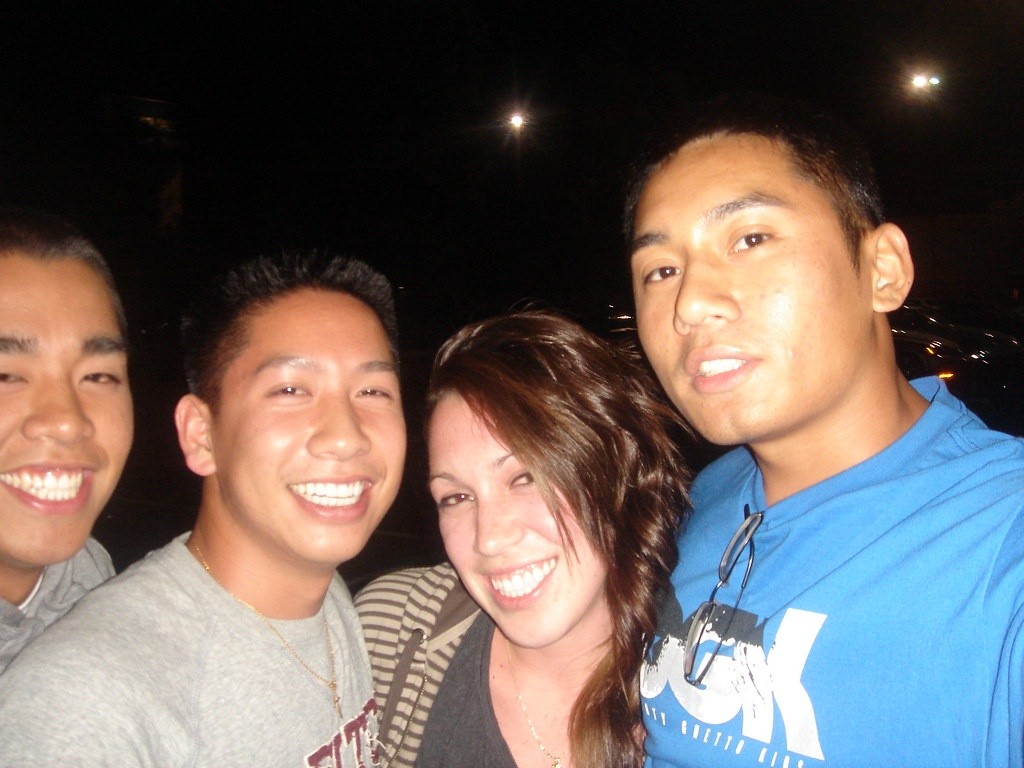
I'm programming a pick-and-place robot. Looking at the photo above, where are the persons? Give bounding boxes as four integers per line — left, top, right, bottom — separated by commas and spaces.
353, 92, 1024, 768
0, 211, 136, 676
0, 251, 406, 768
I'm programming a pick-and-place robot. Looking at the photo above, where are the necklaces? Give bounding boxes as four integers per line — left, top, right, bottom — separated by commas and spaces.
502, 635, 572, 768
192, 531, 343, 719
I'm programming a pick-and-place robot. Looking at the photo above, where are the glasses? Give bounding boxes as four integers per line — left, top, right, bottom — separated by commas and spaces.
681, 502, 766, 688
